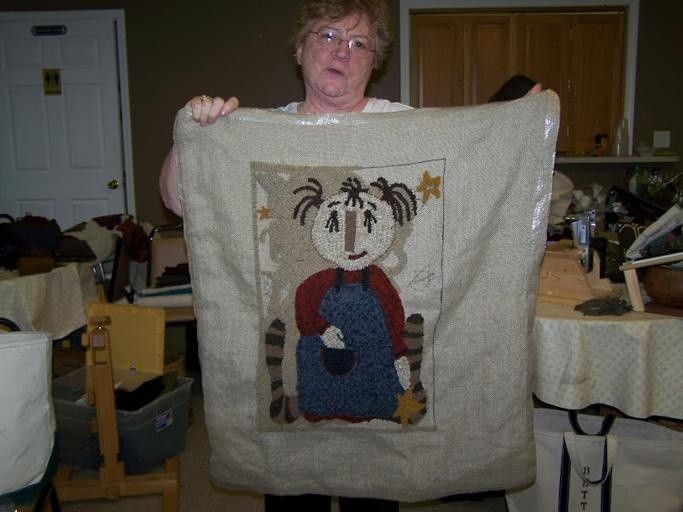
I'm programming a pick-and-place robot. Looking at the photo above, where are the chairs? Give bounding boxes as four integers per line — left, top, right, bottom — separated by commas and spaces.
45, 221, 202, 510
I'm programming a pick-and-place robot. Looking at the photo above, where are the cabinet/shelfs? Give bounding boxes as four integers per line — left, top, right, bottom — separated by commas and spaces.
409, 10, 625, 156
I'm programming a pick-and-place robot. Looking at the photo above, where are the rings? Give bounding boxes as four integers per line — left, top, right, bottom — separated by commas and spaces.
200, 94, 211, 103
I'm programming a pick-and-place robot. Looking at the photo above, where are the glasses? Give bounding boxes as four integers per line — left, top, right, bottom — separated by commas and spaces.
308, 30, 376, 58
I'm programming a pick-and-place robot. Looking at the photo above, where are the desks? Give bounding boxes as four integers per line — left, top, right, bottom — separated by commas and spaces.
525, 242, 683, 434
0, 252, 104, 380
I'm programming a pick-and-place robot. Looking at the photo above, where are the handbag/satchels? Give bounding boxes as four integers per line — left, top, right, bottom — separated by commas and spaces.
1, 318, 57, 496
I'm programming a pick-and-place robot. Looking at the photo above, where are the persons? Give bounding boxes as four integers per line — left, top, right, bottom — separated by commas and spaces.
157, 0, 541, 512
